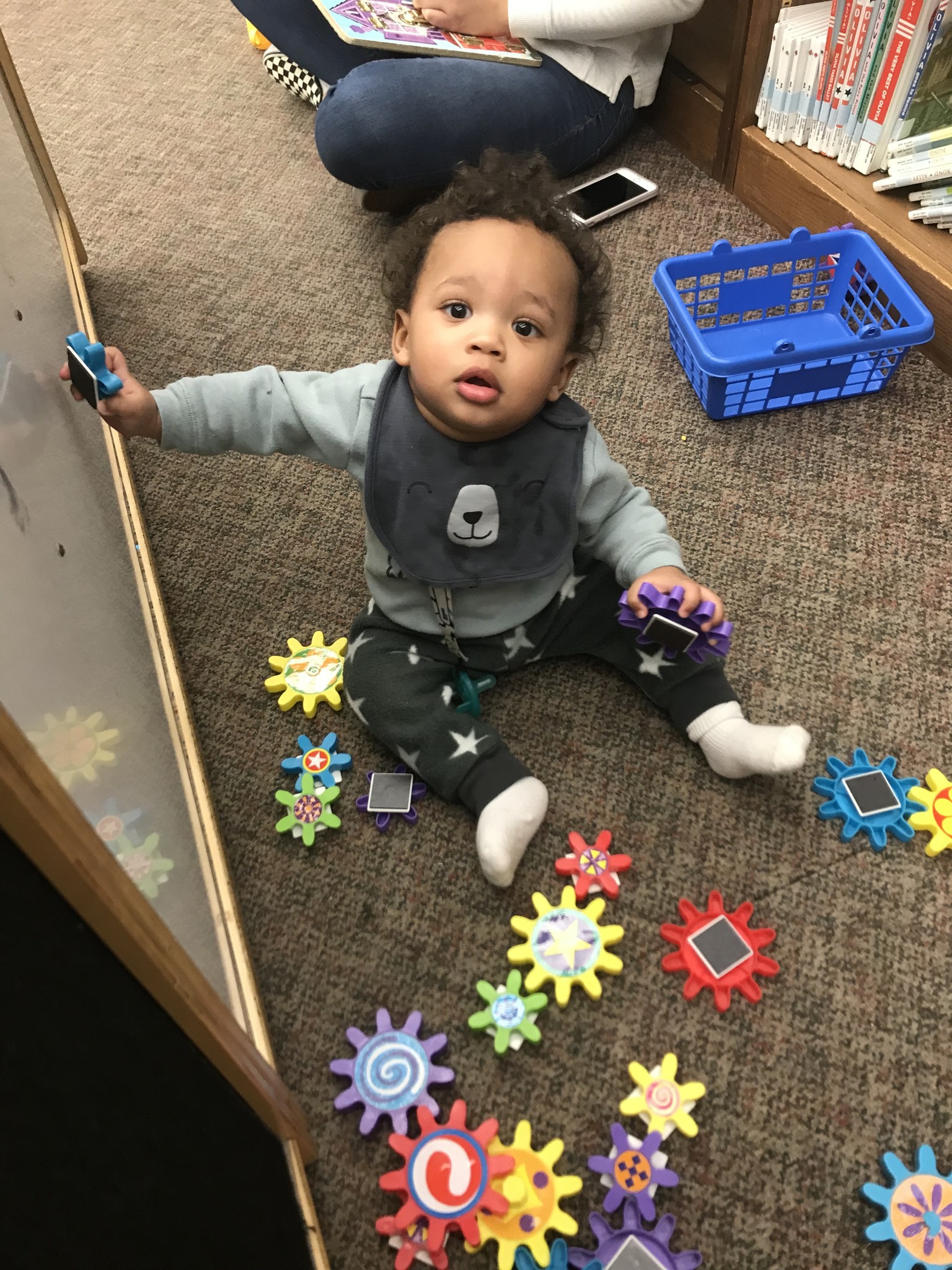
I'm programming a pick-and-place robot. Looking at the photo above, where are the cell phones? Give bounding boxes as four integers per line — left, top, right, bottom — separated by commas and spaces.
554, 167, 661, 227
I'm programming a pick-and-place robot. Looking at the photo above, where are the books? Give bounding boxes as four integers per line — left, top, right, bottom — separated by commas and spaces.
755, 0, 952, 234
312, 0, 543, 67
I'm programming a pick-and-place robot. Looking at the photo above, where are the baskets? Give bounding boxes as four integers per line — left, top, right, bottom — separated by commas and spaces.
656, 232, 937, 423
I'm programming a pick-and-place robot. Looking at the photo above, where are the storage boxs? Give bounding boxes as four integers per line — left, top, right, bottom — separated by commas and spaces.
651, 230, 940, 420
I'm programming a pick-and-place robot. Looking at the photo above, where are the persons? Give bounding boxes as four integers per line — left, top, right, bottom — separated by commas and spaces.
231, 0, 705, 222
59, 147, 810, 887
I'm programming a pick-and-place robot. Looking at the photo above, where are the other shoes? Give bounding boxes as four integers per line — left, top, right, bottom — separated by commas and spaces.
361, 176, 445, 213
264, 44, 334, 111
